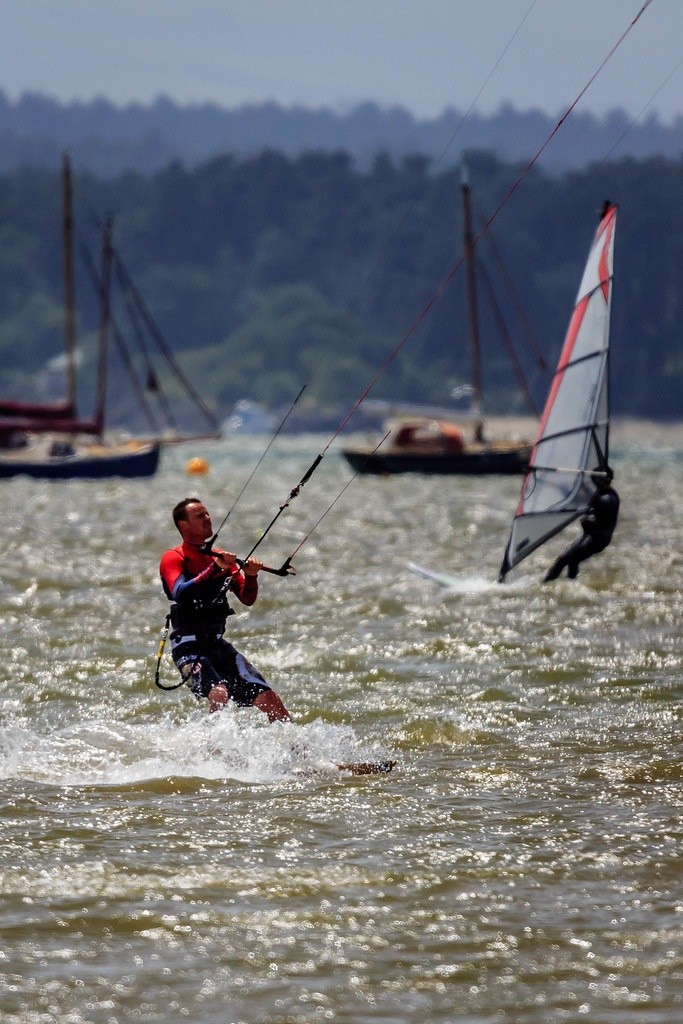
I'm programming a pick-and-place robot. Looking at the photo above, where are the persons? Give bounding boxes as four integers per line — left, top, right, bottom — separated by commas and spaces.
159, 498, 340, 770
541, 467, 620, 583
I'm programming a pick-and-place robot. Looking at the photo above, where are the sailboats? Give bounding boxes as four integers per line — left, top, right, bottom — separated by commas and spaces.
497, 198, 620, 584
0, 147, 223, 478
341, 184, 542, 475
